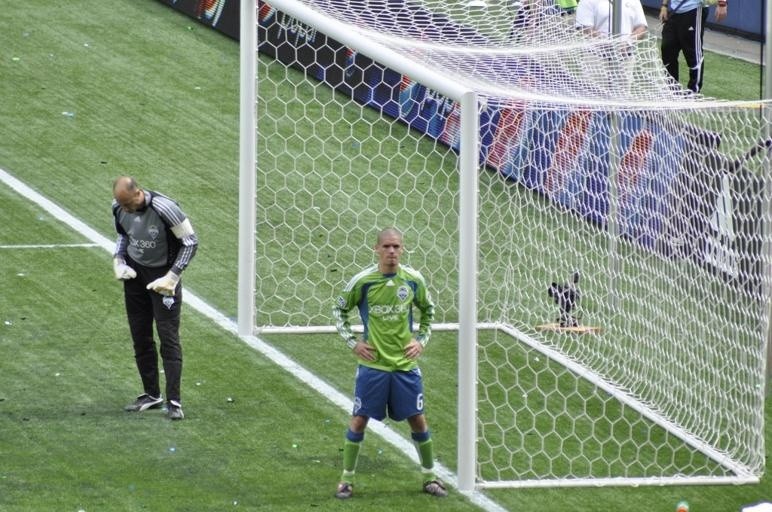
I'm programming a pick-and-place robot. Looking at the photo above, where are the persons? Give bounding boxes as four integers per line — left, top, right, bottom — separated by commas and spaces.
658, 1, 729, 101
573, 0, 650, 93
330, 227, 449, 499
108, 174, 201, 422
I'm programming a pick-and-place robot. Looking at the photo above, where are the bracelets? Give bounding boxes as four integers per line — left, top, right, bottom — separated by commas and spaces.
718, 1, 727, 6
661, 3, 668, 9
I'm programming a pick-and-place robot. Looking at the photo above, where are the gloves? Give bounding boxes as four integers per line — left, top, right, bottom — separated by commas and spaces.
146, 270, 179, 298
113, 258, 137, 280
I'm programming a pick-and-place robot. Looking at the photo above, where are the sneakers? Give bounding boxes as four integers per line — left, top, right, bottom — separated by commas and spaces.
166, 400, 185, 420
335, 481, 354, 499
123, 393, 164, 414
422, 478, 450, 497
